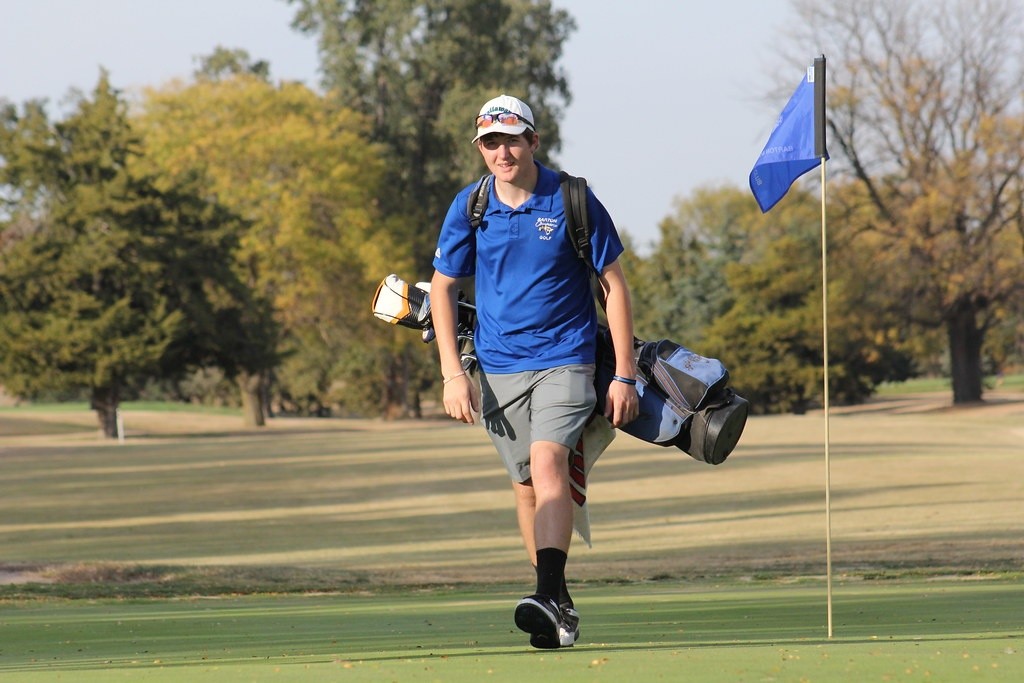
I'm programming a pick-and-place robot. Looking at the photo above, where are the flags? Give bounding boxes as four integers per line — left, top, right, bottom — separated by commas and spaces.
749, 57, 828, 214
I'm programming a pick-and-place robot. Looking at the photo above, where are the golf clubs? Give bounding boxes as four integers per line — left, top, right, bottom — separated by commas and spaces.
417, 294, 476, 326
421, 326, 474, 344
458, 352, 477, 371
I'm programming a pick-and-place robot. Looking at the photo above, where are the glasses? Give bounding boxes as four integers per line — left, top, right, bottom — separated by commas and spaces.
475, 112, 535, 131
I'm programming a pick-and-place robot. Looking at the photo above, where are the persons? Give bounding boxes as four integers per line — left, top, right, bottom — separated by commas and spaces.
430, 95, 639, 649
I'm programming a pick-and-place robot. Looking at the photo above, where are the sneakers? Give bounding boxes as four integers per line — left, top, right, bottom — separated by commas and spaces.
514, 593, 560, 648
558, 602, 580, 646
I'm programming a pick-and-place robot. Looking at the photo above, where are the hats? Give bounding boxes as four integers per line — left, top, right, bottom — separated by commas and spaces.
471, 94, 536, 144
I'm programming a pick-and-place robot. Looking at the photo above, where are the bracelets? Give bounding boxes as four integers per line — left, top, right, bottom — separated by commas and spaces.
443, 371, 465, 383
613, 376, 636, 385
